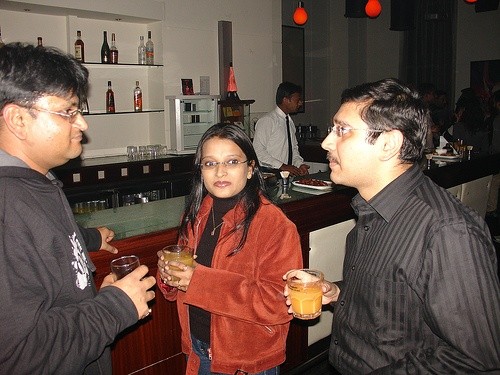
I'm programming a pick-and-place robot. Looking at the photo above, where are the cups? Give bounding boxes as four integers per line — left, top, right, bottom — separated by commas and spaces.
162, 244, 194, 282
71, 200, 106, 215
286, 268, 325, 320
127, 144, 168, 162
110, 255, 140, 282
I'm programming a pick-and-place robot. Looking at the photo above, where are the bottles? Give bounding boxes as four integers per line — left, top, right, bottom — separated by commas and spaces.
138, 36, 147, 64
134, 80, 142, 112
101, 31, 111, 64
146, 31, 154, 65
36, 36, 44, 48
81, 99, 89, 115
110, 33, 119, 64
105, 80, 115, 113
0, 26, 5, 49
443, 138, 466, 158
75, 31, 85, 63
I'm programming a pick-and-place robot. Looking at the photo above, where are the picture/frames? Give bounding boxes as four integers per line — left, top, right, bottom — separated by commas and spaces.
181, 78, 194, 95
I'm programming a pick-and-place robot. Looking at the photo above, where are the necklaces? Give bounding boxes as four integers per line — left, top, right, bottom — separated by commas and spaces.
211, 206, 223, 236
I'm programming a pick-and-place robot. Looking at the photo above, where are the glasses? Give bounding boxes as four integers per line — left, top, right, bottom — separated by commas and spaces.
197, 158, 249, 170
17, 103, 85, 123
285, 116, 293, 165
327, 125, 385, 138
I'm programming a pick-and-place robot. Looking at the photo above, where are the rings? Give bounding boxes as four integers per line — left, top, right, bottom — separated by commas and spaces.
178, 280, 181, 287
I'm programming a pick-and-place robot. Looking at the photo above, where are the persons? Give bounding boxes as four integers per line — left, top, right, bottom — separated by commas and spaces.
252, 82, 309, 178
0, 40, 155, 375
156, 123, 302, 375
282, 80, 500, 375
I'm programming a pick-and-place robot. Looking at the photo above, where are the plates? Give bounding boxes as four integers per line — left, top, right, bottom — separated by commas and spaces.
292, 179, 333, 190
292, 186, 332, 196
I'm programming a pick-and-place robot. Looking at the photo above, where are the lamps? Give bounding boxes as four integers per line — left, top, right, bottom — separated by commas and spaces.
364, 0, 382, 18
292, 1, 309, 26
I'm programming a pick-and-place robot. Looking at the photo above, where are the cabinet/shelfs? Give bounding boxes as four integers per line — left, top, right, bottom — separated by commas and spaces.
81, 63, 164, 116
216, 99, 256, 139
166, 95, 218, 152
